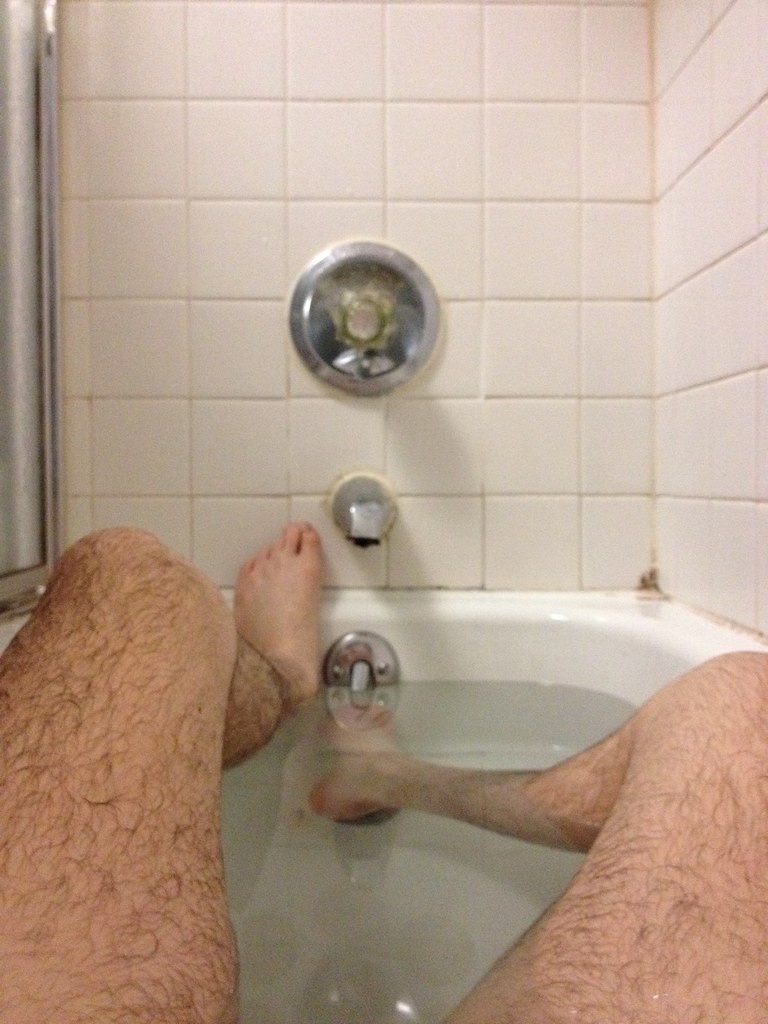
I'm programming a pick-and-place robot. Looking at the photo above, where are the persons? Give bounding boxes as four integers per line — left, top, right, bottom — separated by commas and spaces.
0, 523, 768, 1024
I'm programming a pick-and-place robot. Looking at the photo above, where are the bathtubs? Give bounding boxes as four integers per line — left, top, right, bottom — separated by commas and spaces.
0, 590, 767, 1023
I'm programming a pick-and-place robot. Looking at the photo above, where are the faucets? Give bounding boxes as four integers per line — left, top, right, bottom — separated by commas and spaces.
333, 477, 392, 543
